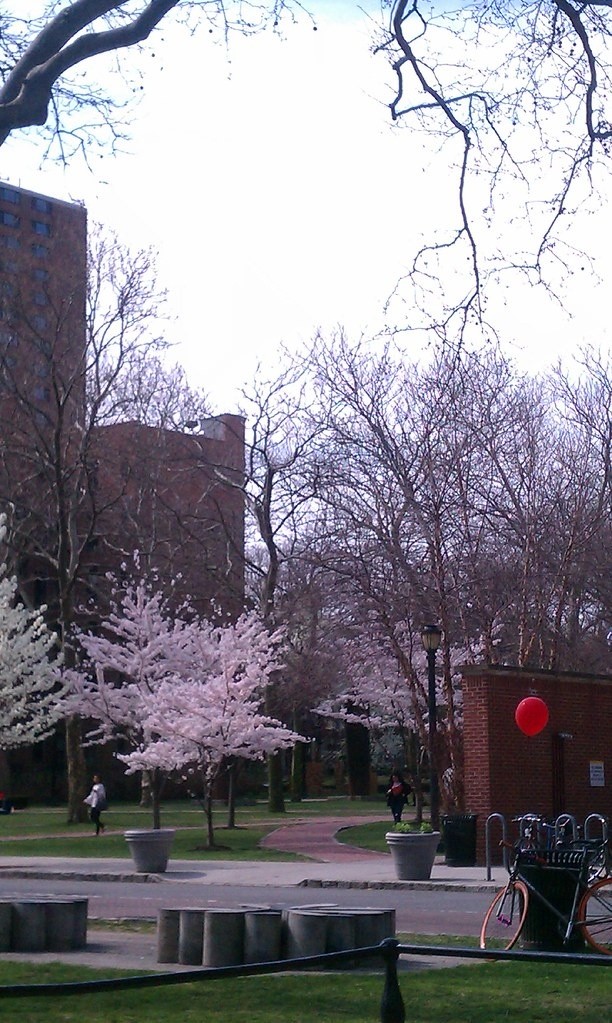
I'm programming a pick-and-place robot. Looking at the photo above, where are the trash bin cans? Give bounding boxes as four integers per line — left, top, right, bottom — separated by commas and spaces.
439, 814, 479, 867
519, 848, 588, 951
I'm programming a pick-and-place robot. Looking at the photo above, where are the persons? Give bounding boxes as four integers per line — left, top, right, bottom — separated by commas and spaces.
82, 773, 106, 835
441, 763, 453, 793
386, 772, 413, 824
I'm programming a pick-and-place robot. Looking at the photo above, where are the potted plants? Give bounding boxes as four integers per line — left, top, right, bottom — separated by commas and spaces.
387, 821, 441, 880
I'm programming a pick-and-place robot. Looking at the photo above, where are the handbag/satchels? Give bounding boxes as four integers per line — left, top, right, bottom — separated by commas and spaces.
95, 793, 108, 812
386, 792, 395, 806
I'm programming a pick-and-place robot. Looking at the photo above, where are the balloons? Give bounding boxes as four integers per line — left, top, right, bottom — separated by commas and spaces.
515, 696, 550, 736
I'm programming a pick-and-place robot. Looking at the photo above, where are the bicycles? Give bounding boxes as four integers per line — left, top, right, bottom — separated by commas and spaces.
505, 814, 612, 884
480, 839, 612, 962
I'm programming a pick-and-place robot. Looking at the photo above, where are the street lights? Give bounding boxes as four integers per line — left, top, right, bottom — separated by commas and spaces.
420, 624, 442, 832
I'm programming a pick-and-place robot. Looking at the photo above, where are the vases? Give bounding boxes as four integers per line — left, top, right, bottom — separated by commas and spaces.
123, 828, 174, 873
